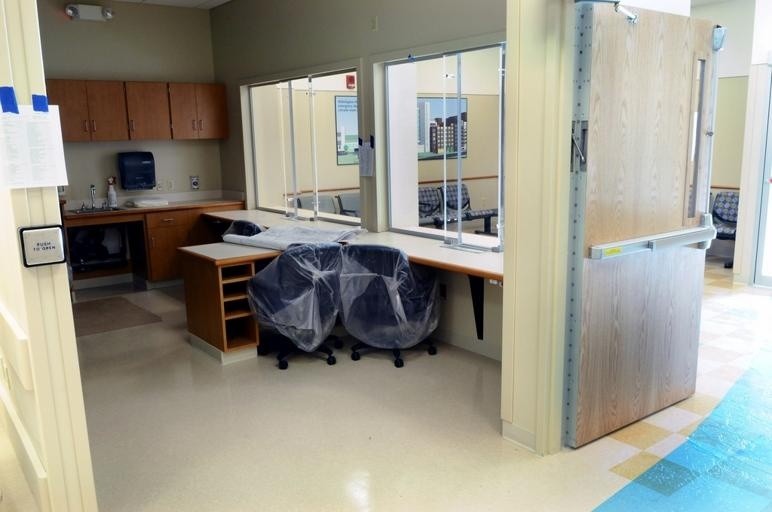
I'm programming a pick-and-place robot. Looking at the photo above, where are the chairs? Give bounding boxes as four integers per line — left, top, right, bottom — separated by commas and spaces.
704, 191, 739, 268
291, 192, 362, 219
418, 183, 500, 237
249, 237, 439, 364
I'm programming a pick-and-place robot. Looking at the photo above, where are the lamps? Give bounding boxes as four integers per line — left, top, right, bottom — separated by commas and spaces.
66, 2, 116, 23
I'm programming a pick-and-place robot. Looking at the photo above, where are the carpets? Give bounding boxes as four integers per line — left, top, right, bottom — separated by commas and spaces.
71, 297, 164, 337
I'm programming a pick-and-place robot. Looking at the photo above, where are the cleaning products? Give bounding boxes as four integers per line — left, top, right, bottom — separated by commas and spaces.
107, 175, 118, 208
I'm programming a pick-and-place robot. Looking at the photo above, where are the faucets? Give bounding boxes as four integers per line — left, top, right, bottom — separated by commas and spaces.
90, 184, 96, 208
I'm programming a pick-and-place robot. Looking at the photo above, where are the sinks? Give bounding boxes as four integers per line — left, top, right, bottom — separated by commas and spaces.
67, 209, 95, 213
94, 206, 128, 212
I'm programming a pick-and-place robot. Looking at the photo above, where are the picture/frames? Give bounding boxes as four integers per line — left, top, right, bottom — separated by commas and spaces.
333, 95, 469, 165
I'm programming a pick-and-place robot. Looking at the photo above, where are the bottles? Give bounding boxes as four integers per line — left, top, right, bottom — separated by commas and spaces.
106, 185, 118, 207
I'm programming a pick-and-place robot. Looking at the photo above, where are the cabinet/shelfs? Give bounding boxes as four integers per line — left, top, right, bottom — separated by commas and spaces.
45, 78, 230, 142
141, 203, 246, 284
178, 244, 285, 353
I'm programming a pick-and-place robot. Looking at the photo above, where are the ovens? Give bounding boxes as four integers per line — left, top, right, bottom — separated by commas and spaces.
66, 220, 149, 294
